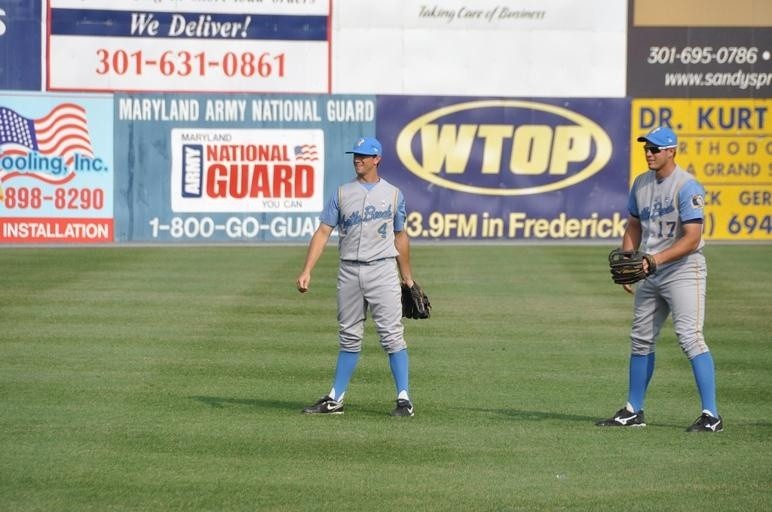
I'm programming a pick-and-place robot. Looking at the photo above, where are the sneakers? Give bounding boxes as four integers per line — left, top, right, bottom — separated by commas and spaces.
597, 406, 645, 427
301, 394, 344, 414
389, 398, 414, 417
685, 413, 723, 432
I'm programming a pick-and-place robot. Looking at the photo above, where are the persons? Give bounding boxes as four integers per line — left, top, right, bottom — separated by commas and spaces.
296, 134, 432, 418
595, 125, 724, 434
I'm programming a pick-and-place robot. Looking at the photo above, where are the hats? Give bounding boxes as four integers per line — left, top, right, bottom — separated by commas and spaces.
637, 127, 677, 147
345, 136, 383, 157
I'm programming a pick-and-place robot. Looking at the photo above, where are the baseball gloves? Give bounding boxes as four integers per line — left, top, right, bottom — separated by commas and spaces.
401, 280, 432, 320
609, 248, 656, 285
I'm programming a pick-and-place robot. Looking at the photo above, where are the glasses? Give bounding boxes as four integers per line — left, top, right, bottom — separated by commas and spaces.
644, 145, 678, 154
